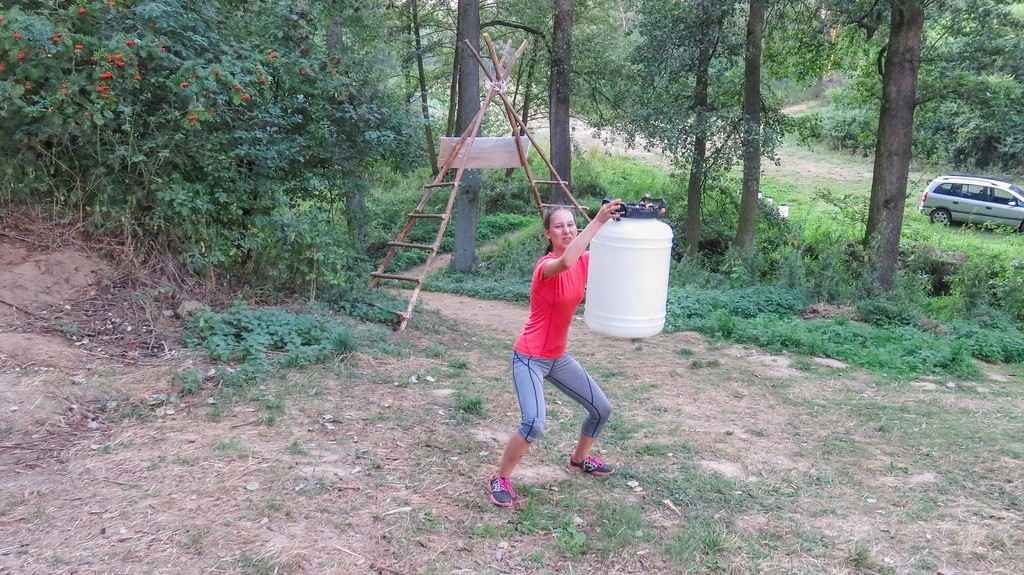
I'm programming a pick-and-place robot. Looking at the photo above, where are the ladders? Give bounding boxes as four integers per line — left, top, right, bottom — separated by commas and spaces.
367, 32, 594, 333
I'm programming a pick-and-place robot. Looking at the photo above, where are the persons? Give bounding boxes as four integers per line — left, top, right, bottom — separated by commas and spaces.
487, 199, 665, 507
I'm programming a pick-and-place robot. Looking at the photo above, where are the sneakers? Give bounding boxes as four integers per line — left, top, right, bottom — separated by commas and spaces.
488, 471, 516, 507
567, 452, 616, 476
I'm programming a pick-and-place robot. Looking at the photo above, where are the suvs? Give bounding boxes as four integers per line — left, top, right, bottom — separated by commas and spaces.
919, 173, 1024, 235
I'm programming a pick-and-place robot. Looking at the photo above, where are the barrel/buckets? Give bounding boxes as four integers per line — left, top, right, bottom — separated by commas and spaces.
584, 199, 674, 337
778, 204, 789, 218
